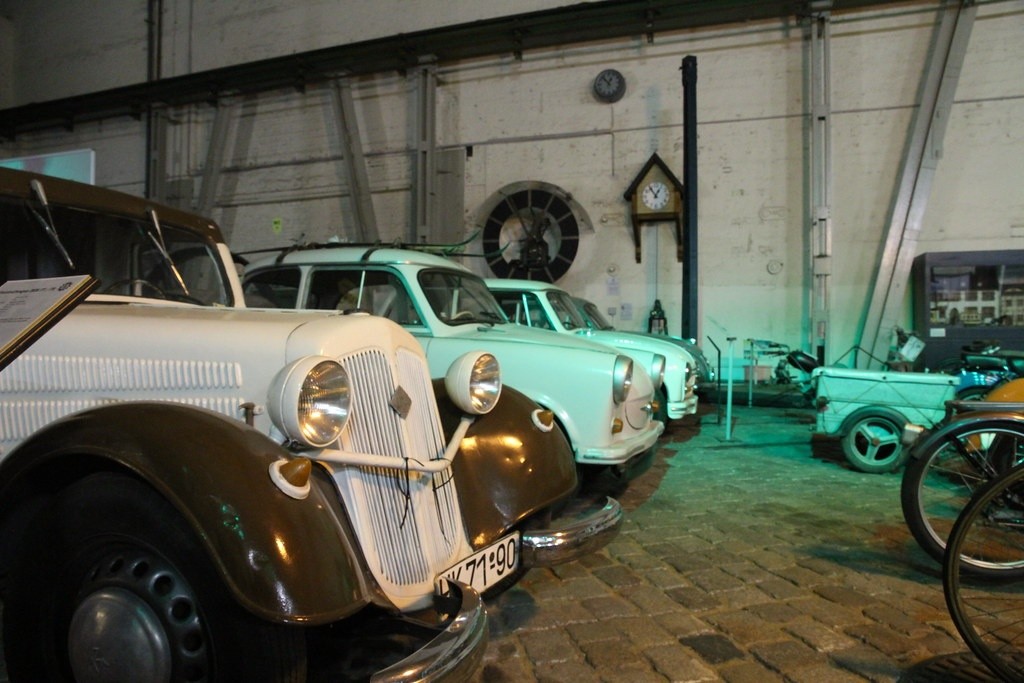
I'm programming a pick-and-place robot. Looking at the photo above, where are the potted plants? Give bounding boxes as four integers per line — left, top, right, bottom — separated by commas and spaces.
743, 338, 789, 381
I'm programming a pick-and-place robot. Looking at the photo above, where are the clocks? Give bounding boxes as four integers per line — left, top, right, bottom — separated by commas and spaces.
642, 181, 671, 211
593, 69, 626, 103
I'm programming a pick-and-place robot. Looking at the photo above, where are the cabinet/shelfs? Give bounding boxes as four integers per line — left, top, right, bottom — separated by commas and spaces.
912, 249, 1024, 372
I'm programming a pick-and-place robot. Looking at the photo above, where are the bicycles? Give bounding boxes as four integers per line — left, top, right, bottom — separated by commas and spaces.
900, 398, 1024, 583
942, 465, 1024, 683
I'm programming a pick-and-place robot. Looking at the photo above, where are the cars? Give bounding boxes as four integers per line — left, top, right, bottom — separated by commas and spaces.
216, 241, 667, 496
483, 279, 719, 434
0, 161, 621, 683
